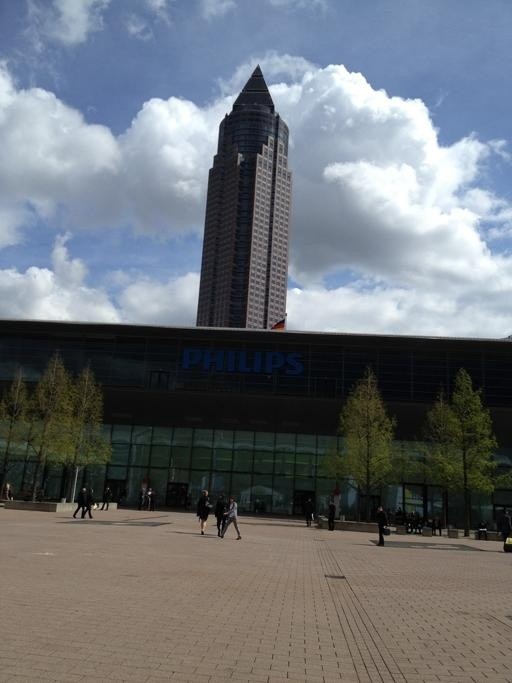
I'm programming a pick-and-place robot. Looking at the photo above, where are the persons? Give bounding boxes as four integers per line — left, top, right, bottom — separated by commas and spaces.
138, 488, 147, 510
99, 486, 113, 511
215, 494, 228, 536
305, 497, 313, 527
220, 496, 242, 540
197, 489, 209, 536
328, 501, 336, 531
146, 488, 155, 511
72, 487, 87, 519
83, 488, 98, 519
376, 505, 387, 546
4, 480, 11, 500
376, 506, 442, 536
478, 510, 512, 542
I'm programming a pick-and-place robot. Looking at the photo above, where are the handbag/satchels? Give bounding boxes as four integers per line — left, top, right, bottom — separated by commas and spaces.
383, 528, 390, 535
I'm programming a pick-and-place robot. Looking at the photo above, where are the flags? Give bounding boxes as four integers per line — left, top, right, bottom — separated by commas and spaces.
271, 318, 285, 330
